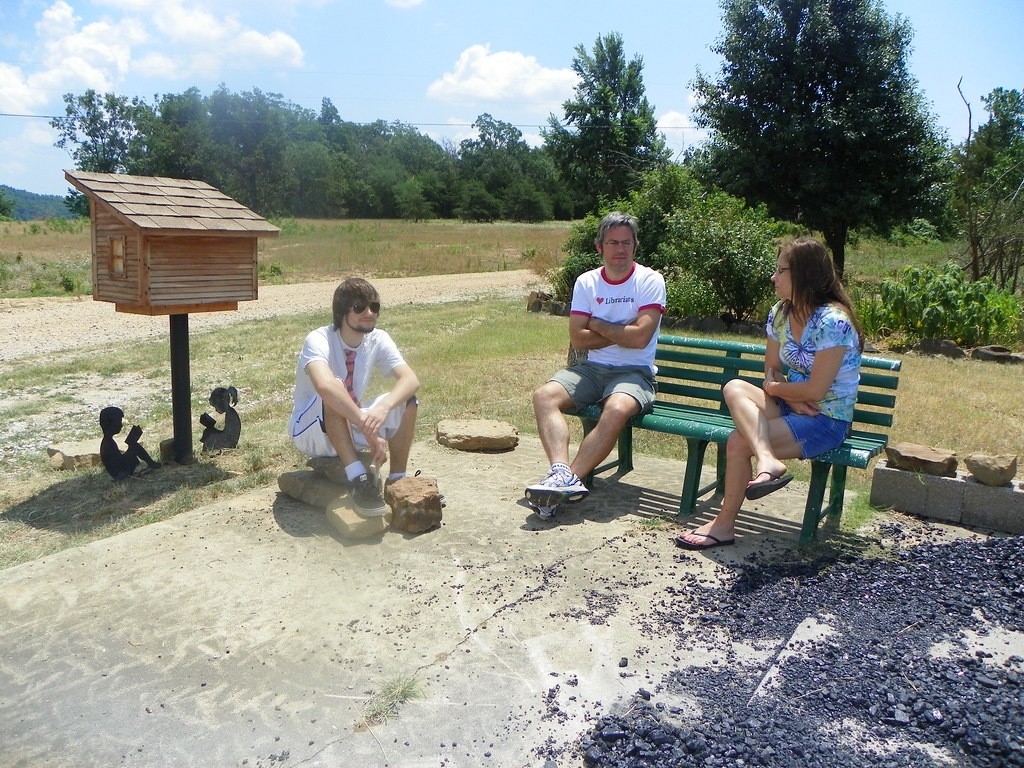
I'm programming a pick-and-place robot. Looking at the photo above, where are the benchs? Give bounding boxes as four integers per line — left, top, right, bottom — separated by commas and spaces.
561, 333, 902, 550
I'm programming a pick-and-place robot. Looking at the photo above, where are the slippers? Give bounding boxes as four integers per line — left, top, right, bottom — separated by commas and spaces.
675, 522, 735, 548
745, 472, 793, 500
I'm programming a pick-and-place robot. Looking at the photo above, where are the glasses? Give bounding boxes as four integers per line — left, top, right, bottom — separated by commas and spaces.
350, 302, 381, 314
775, 264, 790, 274
602, 238, 635, 250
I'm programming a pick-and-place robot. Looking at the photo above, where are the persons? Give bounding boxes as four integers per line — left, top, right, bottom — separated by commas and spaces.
524, 211, 666, 520
675, 238, 866, 549
199, 386, 241, 456
100, 407, 161, 481
288, 279, 421, 516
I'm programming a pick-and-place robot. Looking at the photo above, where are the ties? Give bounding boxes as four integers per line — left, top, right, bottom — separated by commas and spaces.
344, 348, 361, 409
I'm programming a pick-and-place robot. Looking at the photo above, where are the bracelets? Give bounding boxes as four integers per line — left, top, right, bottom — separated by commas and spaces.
765, 379, 773, 394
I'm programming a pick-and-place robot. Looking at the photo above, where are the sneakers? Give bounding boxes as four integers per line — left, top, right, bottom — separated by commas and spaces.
528, 498, 557, 519
525, 467, 589, 507
347, 472, 386, 516
383, 470, 446, 506
